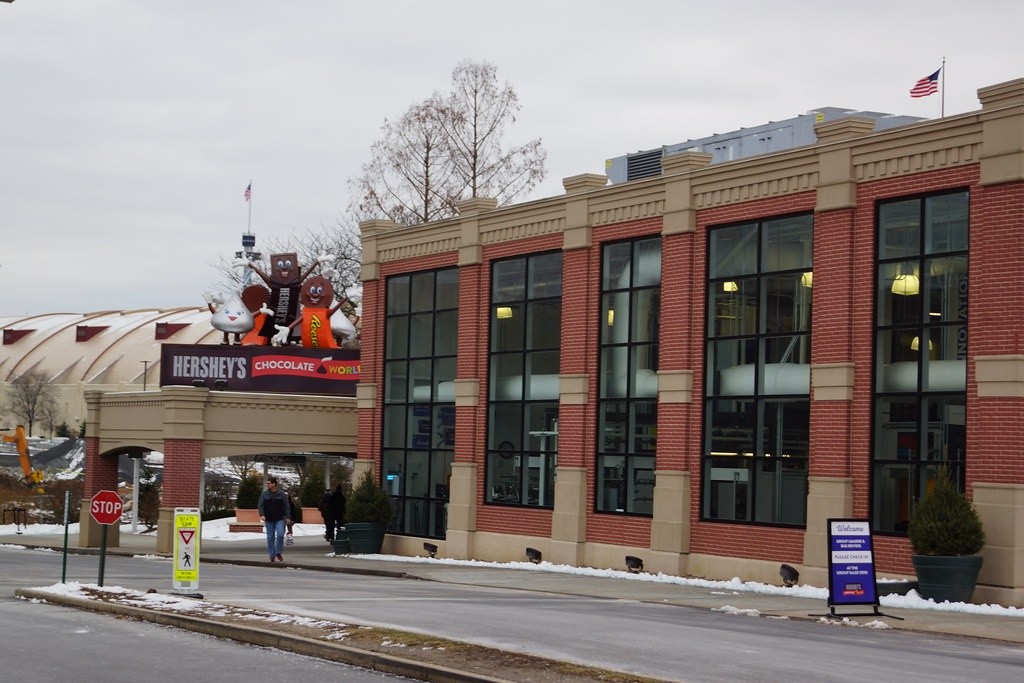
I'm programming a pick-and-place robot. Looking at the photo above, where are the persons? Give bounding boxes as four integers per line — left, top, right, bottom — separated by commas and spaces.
317, 488, 337, 541
258, 476, 291, 562
202, 250, 362, 349
334, 484, 347, 530
286, 492, 296, 545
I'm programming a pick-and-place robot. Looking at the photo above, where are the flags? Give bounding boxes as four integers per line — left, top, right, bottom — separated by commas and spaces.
245, 185, 250, 200
910, 69, 941, 98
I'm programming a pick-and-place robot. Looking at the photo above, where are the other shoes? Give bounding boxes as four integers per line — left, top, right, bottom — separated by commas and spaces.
276, 553, 283, 561
324, 535, 334, 545
270, 557, 274, 562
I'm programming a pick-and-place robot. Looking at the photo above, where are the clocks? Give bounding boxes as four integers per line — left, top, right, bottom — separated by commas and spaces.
498, 441, 515, 459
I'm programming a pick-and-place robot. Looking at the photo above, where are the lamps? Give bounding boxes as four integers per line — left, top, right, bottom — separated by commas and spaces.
424, 542, 439, 557
779, 563, 800, 587
724, 281, 739, 292
801, 272, 813, 288
625, 555, 644, 573
911, 336, 933, 351
526, 548, 542, 563
609, 310, 614, 325
497, 306, 513, 319
891, 264, 919, 295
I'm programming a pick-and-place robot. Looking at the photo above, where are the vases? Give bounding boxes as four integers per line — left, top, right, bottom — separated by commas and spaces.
331, 528, 350, 555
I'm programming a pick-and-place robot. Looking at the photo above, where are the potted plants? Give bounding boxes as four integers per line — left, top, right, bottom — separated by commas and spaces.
909, 464, 986, 604
300, 464, 328, 525
345, 468, 392, 555
234, 475, 265, 521
